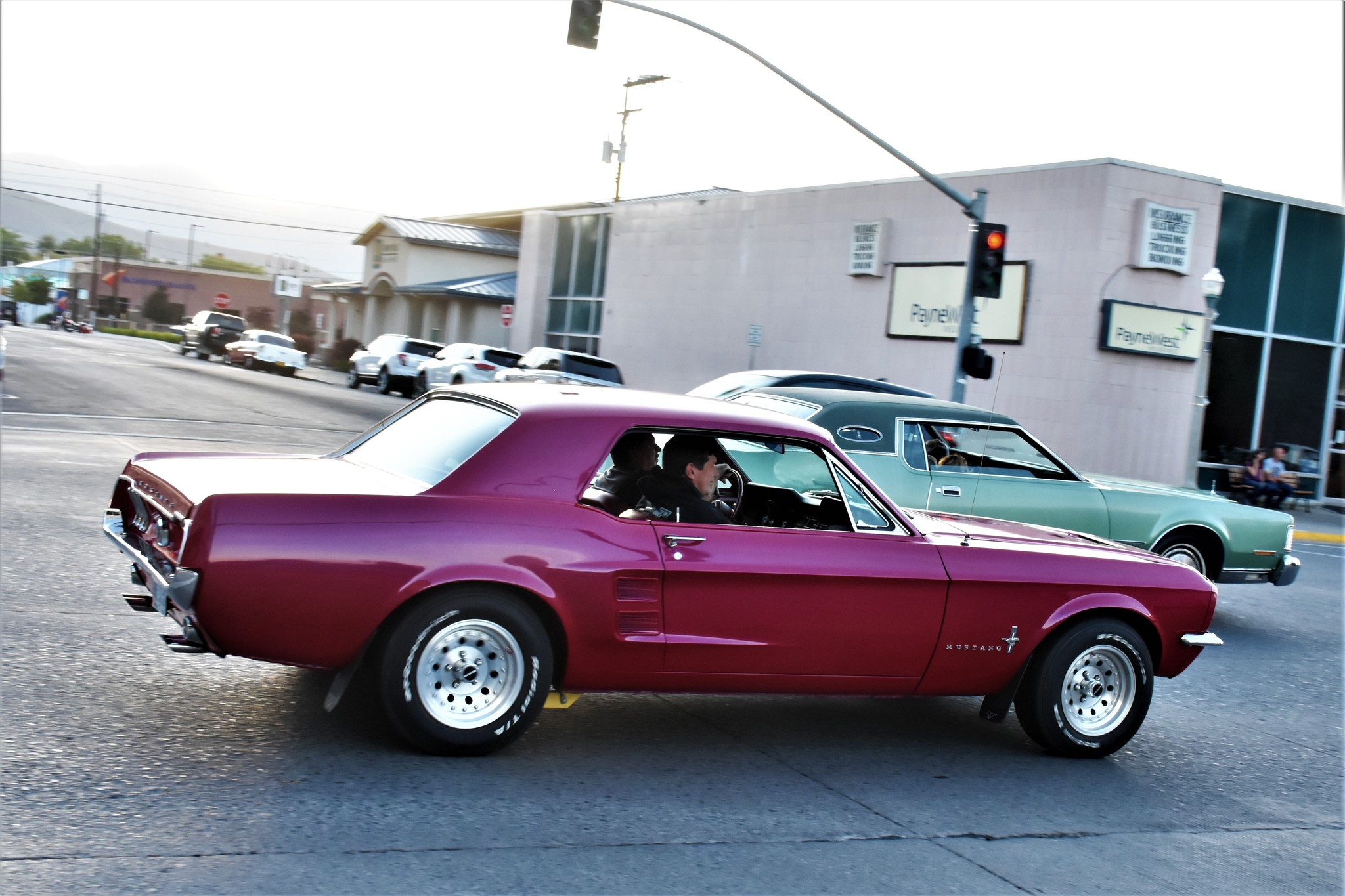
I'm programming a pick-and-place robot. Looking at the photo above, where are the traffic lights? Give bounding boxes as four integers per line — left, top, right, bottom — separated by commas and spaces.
568, 0, 602, 49
969, 222, 1006, 298
961, 346, 993, 378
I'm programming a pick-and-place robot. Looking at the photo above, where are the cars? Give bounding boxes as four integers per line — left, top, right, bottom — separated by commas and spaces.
413, 342, 626, 402
100, 382, 1223, 762
224, 329, 307, 377
598, 370, 1303, 587
0, 323, 6, 381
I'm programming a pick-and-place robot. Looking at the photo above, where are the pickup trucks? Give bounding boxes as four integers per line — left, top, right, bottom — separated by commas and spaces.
1215, 440, 1320, 490
179, 310, 248, 360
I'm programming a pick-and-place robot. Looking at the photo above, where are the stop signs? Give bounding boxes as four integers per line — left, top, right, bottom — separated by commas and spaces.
499, 304, 513, 327
216, 293, 230, 308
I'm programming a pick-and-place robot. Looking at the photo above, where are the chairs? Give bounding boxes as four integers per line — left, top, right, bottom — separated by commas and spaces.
581, 488, 662, 521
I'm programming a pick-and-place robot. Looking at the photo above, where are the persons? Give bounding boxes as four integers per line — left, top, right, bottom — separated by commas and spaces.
595, 431, 734, 501
634, 434, 734, 524
1239, 447, 1295, 511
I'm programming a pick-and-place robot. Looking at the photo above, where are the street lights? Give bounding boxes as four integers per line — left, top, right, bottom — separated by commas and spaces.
1185, 267, 1226, 486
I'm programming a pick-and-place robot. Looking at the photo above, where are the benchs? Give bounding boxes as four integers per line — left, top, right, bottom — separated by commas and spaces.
1228, 467, 1315, 513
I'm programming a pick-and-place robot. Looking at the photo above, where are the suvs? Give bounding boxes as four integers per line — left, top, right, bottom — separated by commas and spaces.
0, 300, 18, 324
345, 334, 445, 399
